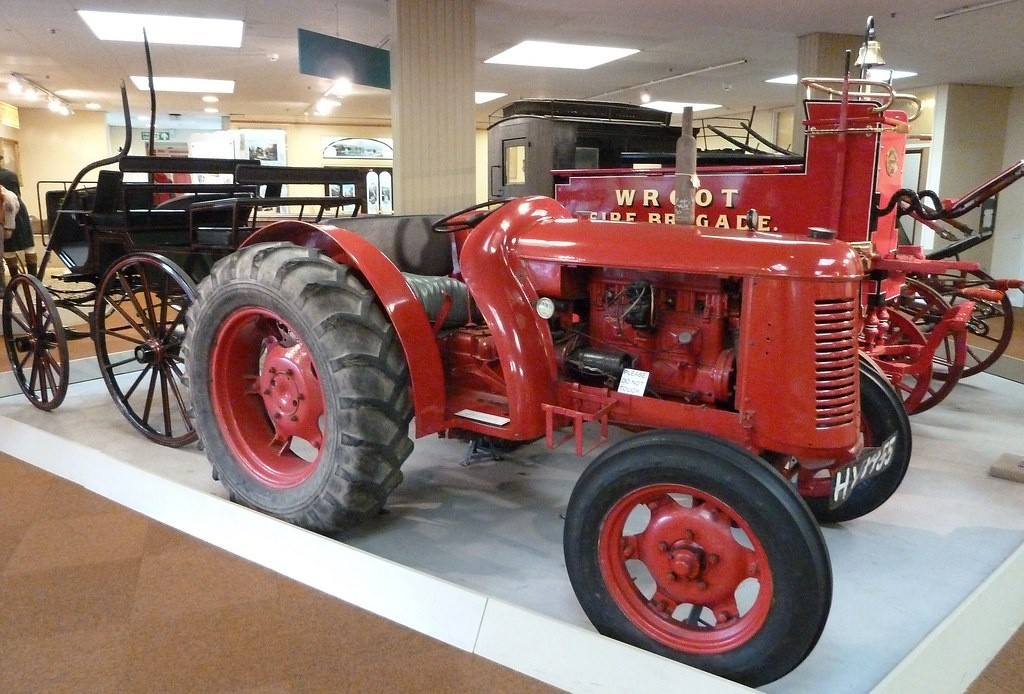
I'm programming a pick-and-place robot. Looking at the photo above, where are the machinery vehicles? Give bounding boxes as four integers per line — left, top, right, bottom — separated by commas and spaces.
180, 16, 912, 691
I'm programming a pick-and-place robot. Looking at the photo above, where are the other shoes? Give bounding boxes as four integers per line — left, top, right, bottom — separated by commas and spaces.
0, 288, 6, 298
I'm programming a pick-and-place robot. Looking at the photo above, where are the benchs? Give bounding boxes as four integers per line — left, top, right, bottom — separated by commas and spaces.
91, 157, 261, 229
197, 164, 363, 247
318, 213, 470, 330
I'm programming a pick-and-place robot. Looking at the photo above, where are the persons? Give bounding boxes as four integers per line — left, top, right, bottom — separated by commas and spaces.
154, 173, 176, 207
0, 155, 37, 301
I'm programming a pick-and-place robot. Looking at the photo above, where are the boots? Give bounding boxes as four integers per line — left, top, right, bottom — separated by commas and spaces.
27, 263, 37, 276
9, 267, 18, 277
